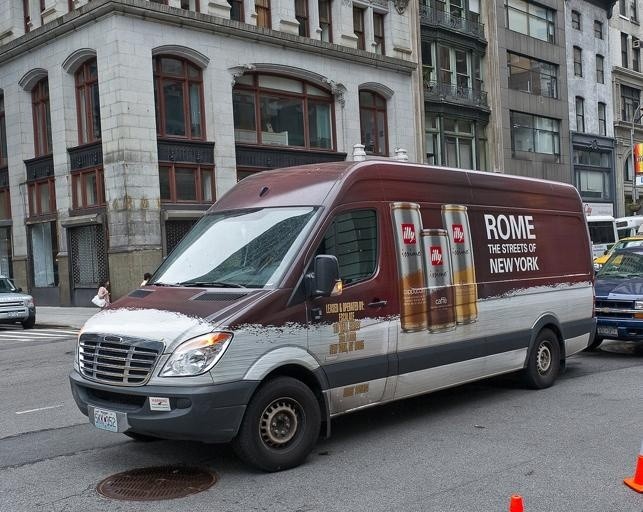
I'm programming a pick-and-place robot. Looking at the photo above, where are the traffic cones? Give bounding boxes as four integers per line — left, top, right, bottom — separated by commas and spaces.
623, 438, 643, 495
508, 492, 528, 511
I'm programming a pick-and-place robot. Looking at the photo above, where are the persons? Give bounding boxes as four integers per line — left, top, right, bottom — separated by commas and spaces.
139, 272, 151, 286
96, 277, 110, 312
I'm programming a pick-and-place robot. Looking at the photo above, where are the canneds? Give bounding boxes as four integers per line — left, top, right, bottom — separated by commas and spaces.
441, 204, 478, 326
389, 201, 428, 331
420, 228, 456, 332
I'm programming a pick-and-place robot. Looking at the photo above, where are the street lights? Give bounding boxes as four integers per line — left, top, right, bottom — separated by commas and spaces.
631, 104, 643, 215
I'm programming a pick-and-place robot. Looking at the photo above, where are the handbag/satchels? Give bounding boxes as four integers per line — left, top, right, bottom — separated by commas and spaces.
91, 294, 106, 308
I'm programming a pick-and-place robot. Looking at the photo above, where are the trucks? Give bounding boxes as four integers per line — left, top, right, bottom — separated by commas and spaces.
70, 158, 600, 474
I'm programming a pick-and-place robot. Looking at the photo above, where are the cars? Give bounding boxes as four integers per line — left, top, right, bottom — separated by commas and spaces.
585, 215, 642, 353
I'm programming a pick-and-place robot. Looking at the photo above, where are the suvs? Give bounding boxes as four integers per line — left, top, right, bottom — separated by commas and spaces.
0, 275, 36, 329
